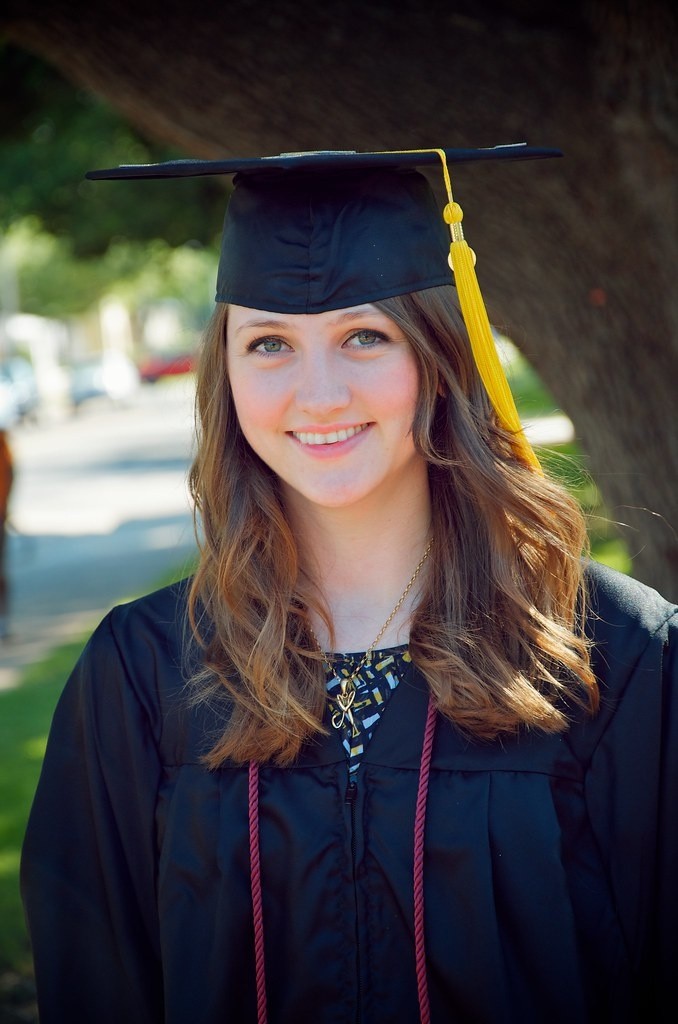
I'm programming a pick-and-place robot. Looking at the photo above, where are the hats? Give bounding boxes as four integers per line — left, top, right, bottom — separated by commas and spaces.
85, 141, 569, 477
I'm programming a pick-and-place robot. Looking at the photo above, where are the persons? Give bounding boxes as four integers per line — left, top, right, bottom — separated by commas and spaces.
19, 141, 678, 1024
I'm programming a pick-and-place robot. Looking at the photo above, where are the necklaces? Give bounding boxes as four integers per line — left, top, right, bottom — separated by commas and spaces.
308, 539, 434, 729
